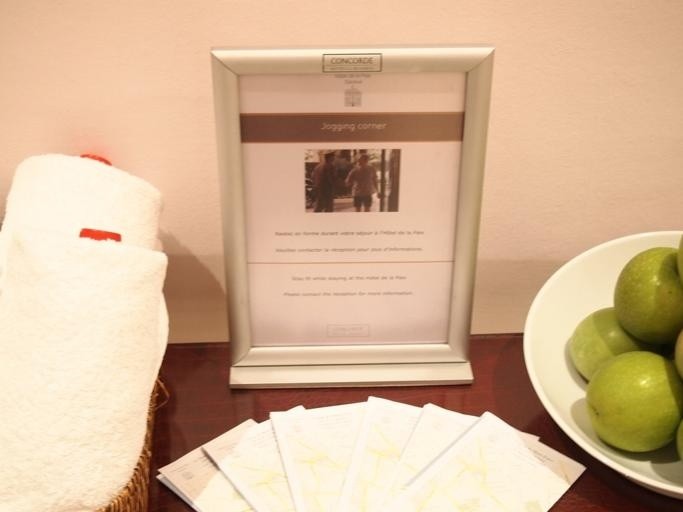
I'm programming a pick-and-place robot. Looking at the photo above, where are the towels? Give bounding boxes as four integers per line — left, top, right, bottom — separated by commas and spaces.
0, 154, 170, 511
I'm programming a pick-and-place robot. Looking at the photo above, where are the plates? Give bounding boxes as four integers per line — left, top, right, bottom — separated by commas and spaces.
522, 228, 683, 501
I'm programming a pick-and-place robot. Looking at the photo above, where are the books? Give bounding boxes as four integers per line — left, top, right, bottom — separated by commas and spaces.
157, 393, 587, 512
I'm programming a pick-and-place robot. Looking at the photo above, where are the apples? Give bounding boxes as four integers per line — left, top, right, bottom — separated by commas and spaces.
613, 247, 683, 345
676, 235, 683, 286
568, 306, 657, 382
675, 419, 683, 460
672, 329, 683, 374
586, 350, 682, 452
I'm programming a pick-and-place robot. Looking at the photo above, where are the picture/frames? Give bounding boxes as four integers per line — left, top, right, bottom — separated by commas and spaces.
208, 44, 495, 367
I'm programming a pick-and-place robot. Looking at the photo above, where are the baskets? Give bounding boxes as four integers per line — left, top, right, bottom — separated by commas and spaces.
91, 381, 155, 512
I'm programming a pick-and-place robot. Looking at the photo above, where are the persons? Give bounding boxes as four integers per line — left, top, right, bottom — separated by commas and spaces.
344, 153, 381, 211
311, 150, 345, 213
